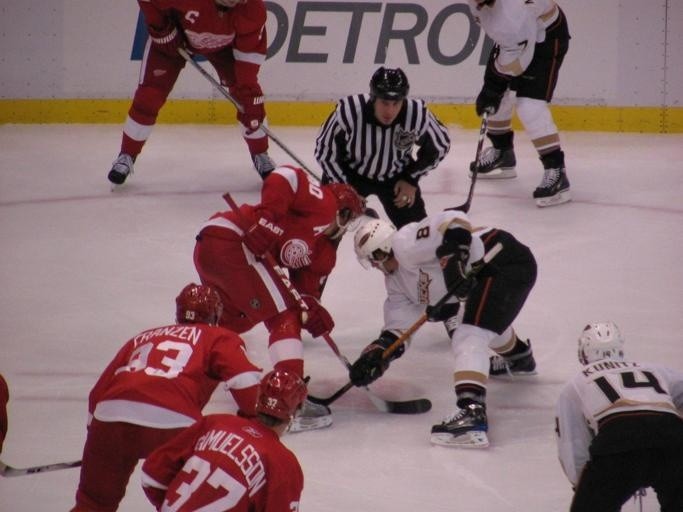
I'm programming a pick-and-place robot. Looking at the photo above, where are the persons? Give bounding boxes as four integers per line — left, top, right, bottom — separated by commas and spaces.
141, 368, 308, 512
348, 211, 538, 449
314, 68, 451, 231
108, 0, 276, 193
556, 321, 682, 511
463, 1, 573, 207
68, 284, 262, 511
192, 163, 363, 432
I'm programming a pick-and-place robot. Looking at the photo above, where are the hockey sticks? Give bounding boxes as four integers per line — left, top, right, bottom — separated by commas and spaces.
177, 48, 379, 218
222, 192, 433, 413
443, 42, 498, 215
305, 241, 505, 406
0, 461, 82, 478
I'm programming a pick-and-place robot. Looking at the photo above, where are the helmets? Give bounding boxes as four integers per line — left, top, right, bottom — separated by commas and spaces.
369, 65, 409, 101
325, 182, 365, 241
257, 365, 309, 422
175, 282, 225, 325
354, 219, 396, 271
577, 321, 626, 366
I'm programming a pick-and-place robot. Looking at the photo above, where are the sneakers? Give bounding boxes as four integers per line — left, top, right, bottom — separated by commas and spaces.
531, 146, 569, 198
430, 393, 490, 435
489, 344, 538, 376
252, 148, 276, 177
469, 140, 518, 171
110, 152, 135, 185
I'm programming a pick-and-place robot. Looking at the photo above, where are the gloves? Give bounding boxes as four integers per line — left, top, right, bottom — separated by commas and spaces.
247, 207, 284, 260
297, 291, 336, 339
348, 330, 406, 386
472, 70, 509, 115
235, 77, 271, 130
436, 229, 475, 297
148, 19, 186, 60
